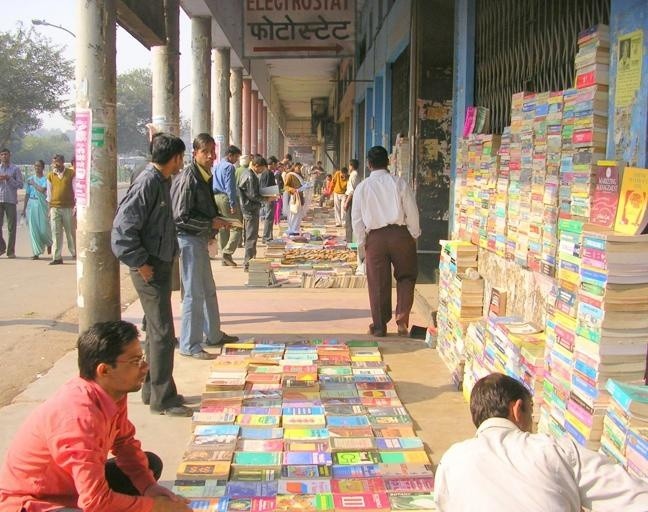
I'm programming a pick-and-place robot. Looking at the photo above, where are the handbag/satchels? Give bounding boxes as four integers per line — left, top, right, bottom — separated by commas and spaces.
289, 190, 300, 214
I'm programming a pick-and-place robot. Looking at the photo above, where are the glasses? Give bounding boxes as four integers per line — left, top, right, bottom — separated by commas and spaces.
102, 355, 147, 368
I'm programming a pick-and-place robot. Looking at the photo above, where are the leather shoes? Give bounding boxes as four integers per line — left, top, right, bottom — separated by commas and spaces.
145, 393, 185, 405
180, 349, 217, 360
151, 402, 193, 418
206, 334, 239, 346
49, 258, 63, 264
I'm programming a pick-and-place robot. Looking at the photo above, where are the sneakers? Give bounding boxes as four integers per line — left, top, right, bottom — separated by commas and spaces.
368, 323, 387, 336
397, 324, 408, 336
223, 254, 236, 266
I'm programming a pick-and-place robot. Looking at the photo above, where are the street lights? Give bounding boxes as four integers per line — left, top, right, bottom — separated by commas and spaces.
31, 18, 76, 40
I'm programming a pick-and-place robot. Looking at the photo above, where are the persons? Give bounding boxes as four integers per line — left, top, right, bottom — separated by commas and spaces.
0, 321, 193, 512
434, 374, 648, 512
